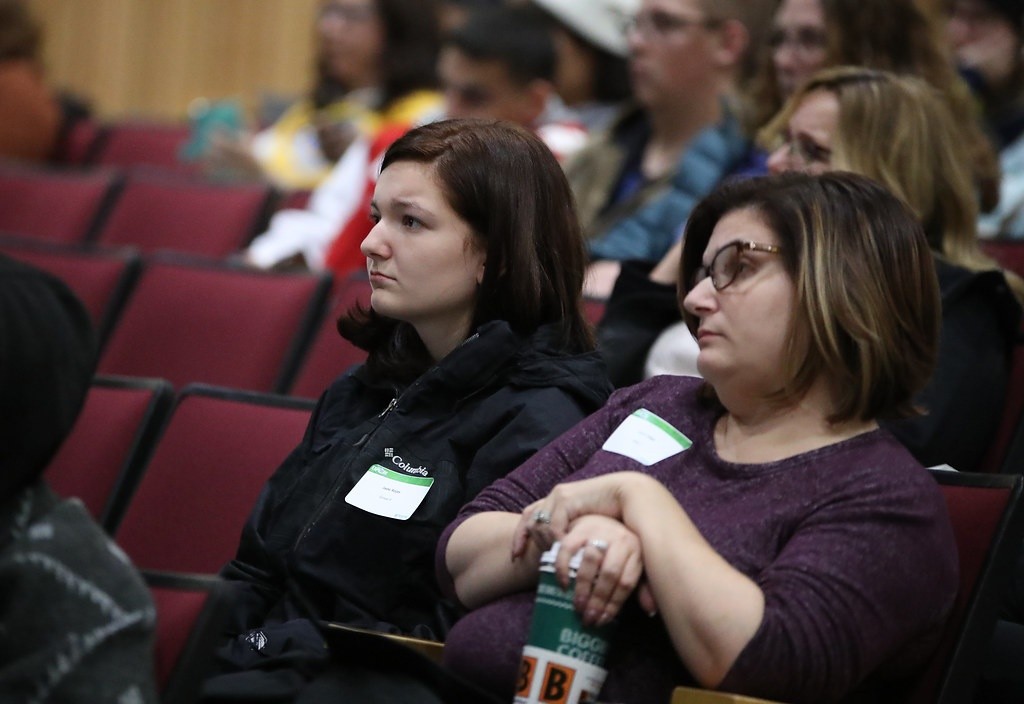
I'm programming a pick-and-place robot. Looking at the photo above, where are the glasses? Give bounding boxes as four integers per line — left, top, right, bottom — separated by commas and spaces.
695, 240, 783, 291
620, 11, 706, 37
776, 128, 832, 167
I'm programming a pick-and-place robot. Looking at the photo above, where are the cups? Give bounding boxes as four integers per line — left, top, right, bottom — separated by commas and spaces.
510, 541, 615, 704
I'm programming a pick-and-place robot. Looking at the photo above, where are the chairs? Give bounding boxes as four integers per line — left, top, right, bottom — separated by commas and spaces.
0, 63, 1022, 704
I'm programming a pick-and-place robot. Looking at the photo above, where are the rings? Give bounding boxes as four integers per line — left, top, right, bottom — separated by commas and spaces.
535, 509, 552, 524
590, 538, 610, 554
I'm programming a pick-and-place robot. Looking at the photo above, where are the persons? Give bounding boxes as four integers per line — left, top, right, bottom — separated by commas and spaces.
0, 0, 127, 183
429, 168, 949, 704
0, 252, 161, 702
188, 1, 1024, 485
161, 115, 616, 704
